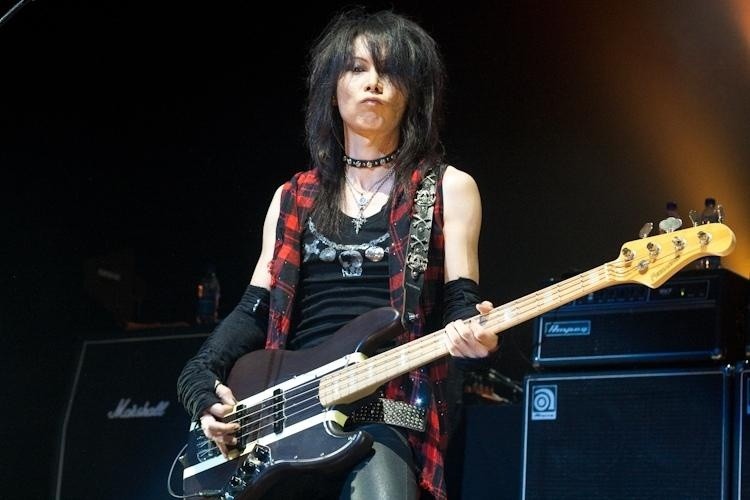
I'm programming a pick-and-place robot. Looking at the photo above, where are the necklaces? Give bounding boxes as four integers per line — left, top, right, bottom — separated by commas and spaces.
343, 168, 396, 235
343, 161, 396, 204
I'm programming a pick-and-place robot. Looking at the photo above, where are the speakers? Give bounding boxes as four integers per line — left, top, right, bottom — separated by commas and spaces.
55, 333, 210, 500
520, 368, 731, 500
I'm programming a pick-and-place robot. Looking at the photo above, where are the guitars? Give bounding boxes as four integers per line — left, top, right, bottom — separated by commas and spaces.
166, 204, 737, 497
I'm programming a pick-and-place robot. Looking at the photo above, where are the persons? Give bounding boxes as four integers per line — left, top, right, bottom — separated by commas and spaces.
172, 6, 501, 499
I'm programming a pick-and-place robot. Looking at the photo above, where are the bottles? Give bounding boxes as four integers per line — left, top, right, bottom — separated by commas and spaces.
193, 263, 220, 333
699, 198, 721, 268
665, 200, 683, 231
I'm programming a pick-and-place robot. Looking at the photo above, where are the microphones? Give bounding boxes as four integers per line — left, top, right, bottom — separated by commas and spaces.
487, 368, 516, 389
